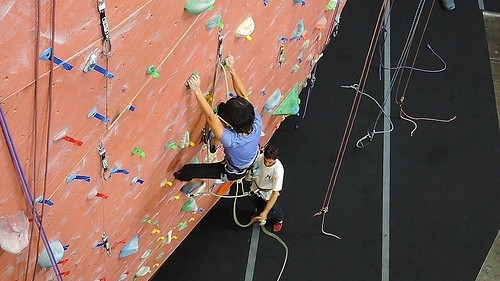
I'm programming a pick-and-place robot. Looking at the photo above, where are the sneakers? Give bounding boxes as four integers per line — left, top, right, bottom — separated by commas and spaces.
273, 223, 283, 232
252, 214, 258, 222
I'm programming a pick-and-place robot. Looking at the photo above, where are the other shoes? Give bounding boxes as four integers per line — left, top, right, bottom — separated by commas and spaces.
173, 171, 192, 181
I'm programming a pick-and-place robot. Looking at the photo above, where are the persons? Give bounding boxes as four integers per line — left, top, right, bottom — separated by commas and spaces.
244, 146, 285, 232
173, 53, 263, 182
